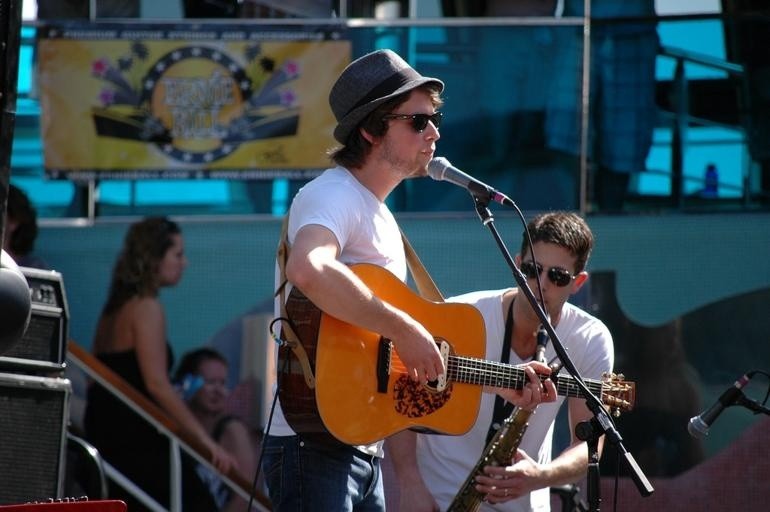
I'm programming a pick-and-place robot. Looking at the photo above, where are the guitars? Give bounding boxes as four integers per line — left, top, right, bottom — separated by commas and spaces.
278, 262, 636, 446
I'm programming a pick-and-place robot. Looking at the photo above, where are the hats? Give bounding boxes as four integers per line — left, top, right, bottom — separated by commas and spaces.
328, 48, 446, 146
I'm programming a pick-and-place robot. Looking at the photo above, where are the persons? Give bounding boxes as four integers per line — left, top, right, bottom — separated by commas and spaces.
176, 348, 256, 511
2, 184, 48, 271
82, 216, 242, 511
261, 49, 558, 511
392, 209, 615, 511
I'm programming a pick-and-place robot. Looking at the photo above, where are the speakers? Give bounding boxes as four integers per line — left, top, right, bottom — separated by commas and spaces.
0, 263, 72, 505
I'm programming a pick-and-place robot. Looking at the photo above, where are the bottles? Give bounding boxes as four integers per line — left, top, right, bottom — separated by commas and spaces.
705, 165, 719, 200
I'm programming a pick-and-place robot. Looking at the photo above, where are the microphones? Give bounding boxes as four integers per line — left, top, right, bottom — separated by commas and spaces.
534, 314, 551, 363
687, 371, 754, 439
427, 157, 512, 210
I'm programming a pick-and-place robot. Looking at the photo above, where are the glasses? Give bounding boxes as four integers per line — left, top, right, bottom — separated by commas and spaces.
520, 259, 580, 288
386, 112, 444, 133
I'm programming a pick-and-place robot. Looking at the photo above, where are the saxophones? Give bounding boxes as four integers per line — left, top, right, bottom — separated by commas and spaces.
447, 316, 550, 512
